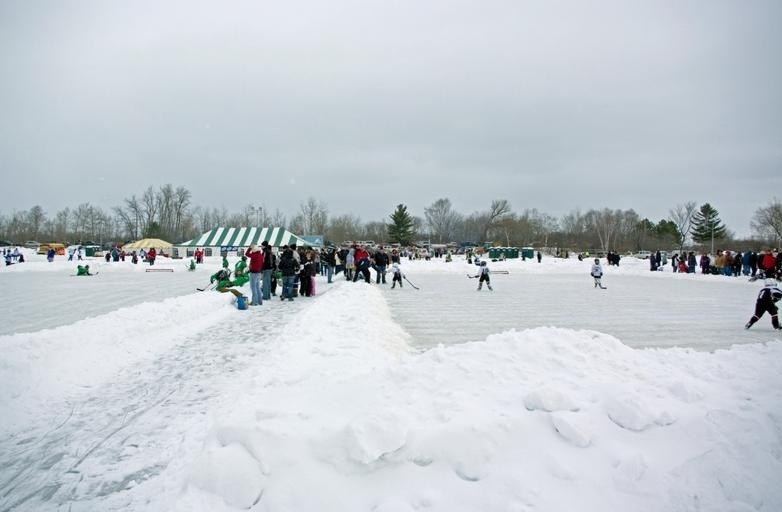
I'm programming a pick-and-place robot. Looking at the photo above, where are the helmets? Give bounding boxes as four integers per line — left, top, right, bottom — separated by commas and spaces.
594, 258, 599, 265
480, 261, 486, 266
392, 262, 398, 268
764, 278, 778, 287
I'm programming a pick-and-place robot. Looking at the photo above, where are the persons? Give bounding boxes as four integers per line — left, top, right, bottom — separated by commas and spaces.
475, 260, 493, 292
47, 247, 57, 262
66, 248, 77, 262
106, 245, 156, 267
743, 277, 781, 330
209, 240, 443, 308
537, 250, 543, 263
200, 248, 204, 264
12, 248, 18, 261
193, 247, 201, 264
590, 258, 603, 288
607, 247, 781, 281
78, 249, 83, 261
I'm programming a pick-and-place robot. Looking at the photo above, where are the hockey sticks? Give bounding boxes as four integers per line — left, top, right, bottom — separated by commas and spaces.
594, 276, 607, 289
197, 280, 216, 291
405, 277, 420, 289
467, 273, 479, 278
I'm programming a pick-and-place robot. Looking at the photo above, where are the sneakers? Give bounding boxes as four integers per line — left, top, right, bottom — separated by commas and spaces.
746, 323, 751, 328
774, 325, 782, 329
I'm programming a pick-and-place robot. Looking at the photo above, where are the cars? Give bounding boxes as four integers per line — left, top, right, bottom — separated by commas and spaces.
635, 250, 653, 260
0, 240, 14, 246
341, 239, 437, 258
83, 241, 98, 246
24, 240, 40, 247
655, 250, 672, 258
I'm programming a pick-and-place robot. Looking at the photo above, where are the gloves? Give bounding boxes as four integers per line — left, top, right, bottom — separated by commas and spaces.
403, 275, 405, 279
591, 272, 594, 276
475, 275, 480, 278
384, 271, 387, 274
600, 272, 603, 276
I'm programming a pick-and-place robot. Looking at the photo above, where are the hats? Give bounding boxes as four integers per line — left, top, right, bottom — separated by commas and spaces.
379, 247, 385, 250
261, 241, 268, 246
252, 245, 260, 253
290, 244, 297, 250
